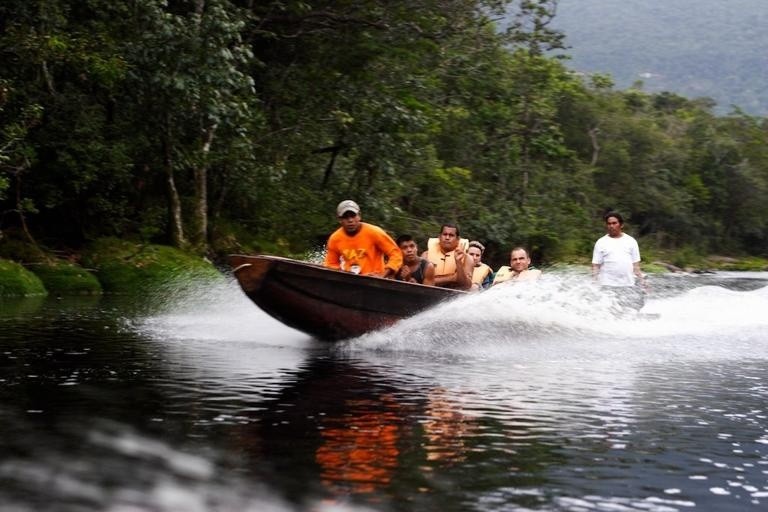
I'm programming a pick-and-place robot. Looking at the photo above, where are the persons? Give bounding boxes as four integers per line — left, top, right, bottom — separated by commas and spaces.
322, 200, 403, 278
395, 222, 541, 295
592, 213, 647, 310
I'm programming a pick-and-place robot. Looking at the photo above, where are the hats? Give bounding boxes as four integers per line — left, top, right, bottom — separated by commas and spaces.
337, 200, 360, 217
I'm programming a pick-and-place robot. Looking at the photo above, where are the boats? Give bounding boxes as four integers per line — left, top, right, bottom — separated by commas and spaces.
231, 251, 466, 345
